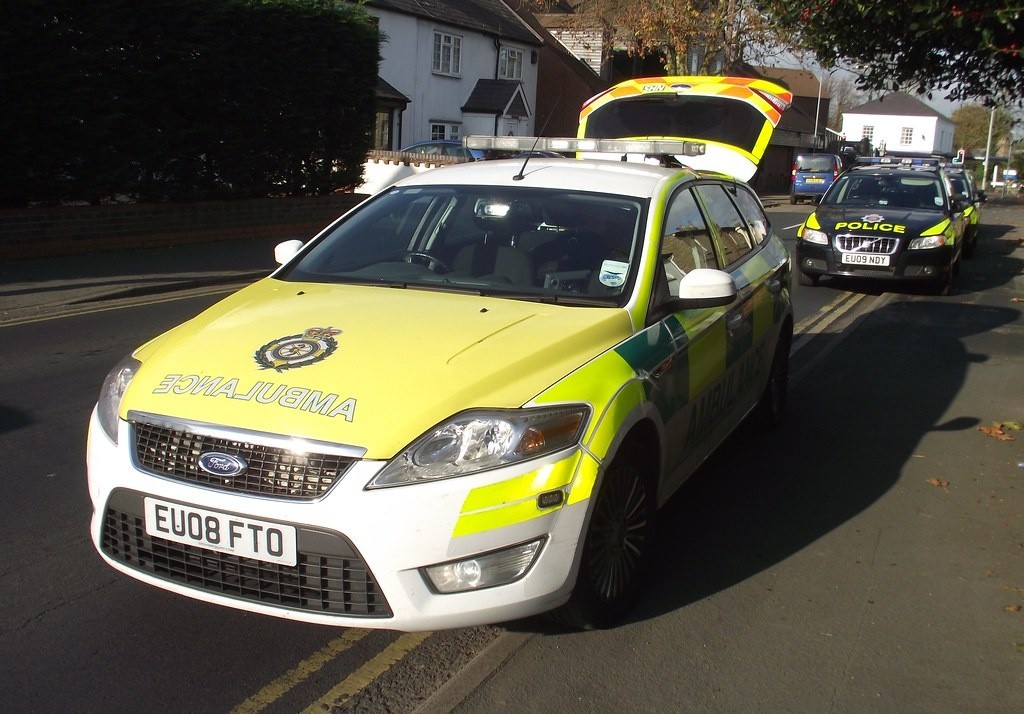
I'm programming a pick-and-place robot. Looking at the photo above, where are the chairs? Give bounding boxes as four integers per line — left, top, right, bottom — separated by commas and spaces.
450, 242, 536, 288
912, 184, 940, 207
854, 177, 881, 198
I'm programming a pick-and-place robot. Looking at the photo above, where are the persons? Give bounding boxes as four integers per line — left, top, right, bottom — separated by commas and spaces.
748, 215, 767, 244
731, 219, 752, 251
875, 140, 887, 157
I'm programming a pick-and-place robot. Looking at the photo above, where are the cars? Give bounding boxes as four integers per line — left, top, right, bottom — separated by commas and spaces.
81, 76, 800, 633
791, 152, 841, 204
400, 139, 563, 164
796, 163, 980, 291
